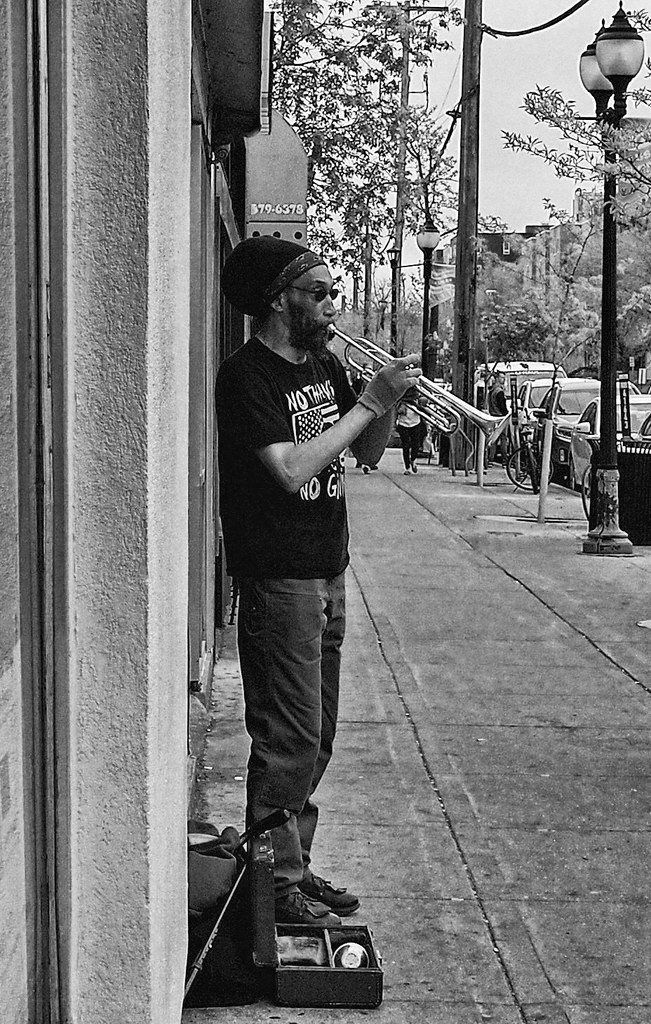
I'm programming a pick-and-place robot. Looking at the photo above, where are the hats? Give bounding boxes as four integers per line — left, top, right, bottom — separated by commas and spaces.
220, 235, 328, 318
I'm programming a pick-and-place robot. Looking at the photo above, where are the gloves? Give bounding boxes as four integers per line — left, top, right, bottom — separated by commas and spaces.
356, 354, 422, 419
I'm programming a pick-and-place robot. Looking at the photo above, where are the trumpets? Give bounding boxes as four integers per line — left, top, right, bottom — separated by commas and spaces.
326, 323, 511, 449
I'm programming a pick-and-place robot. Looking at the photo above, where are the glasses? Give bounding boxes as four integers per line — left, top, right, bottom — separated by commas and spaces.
289, 286, 339, 302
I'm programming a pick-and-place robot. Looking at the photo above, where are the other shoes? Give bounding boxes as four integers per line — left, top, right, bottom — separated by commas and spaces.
404, 470, 409, 475
502, 463, 507, 468
487, 462, 493, 468
410, 462, 418, 473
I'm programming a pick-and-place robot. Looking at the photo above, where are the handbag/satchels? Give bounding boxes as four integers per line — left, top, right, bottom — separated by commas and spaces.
188, 818, 277, 1007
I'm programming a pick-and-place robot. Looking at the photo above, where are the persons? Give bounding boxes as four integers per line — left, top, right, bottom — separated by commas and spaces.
351, 371, 509, 475
214, 235, 421, 930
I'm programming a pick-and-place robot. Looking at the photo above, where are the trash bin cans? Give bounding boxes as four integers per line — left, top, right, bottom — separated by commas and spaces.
585, 437, 651, 547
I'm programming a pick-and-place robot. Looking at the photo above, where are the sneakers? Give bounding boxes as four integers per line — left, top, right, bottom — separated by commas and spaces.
299, 873, 360, 916
273, 891, 342, 926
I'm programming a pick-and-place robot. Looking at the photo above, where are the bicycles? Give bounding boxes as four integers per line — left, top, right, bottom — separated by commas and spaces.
503, 407, 556, 495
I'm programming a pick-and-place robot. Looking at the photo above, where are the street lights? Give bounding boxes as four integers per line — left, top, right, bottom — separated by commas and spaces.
579, 0, 634, 555
411, 207, 437, 458
387, 246, 401, 358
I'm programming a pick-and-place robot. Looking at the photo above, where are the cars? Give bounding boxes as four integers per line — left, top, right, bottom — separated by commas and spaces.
475, 360, 651, 498
